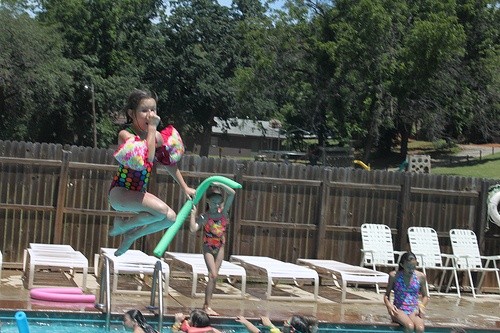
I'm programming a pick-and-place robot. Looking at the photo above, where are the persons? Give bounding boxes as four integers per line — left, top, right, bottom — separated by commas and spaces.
123, 309, 487, 333
384, 252, 428, 333
108, 89, 196, 257
190, 181, 236, 316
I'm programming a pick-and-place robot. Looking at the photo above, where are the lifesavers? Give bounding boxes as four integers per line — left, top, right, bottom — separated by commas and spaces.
30, 287, 95, 302
15, 311, 30, 333
181, 320, 221, 333
488, 191, 500, 226
113, 136, 148, 171
155, 125, 185, 166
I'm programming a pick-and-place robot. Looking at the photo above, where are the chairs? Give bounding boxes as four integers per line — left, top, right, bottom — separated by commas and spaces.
23, 223, 500, 303
